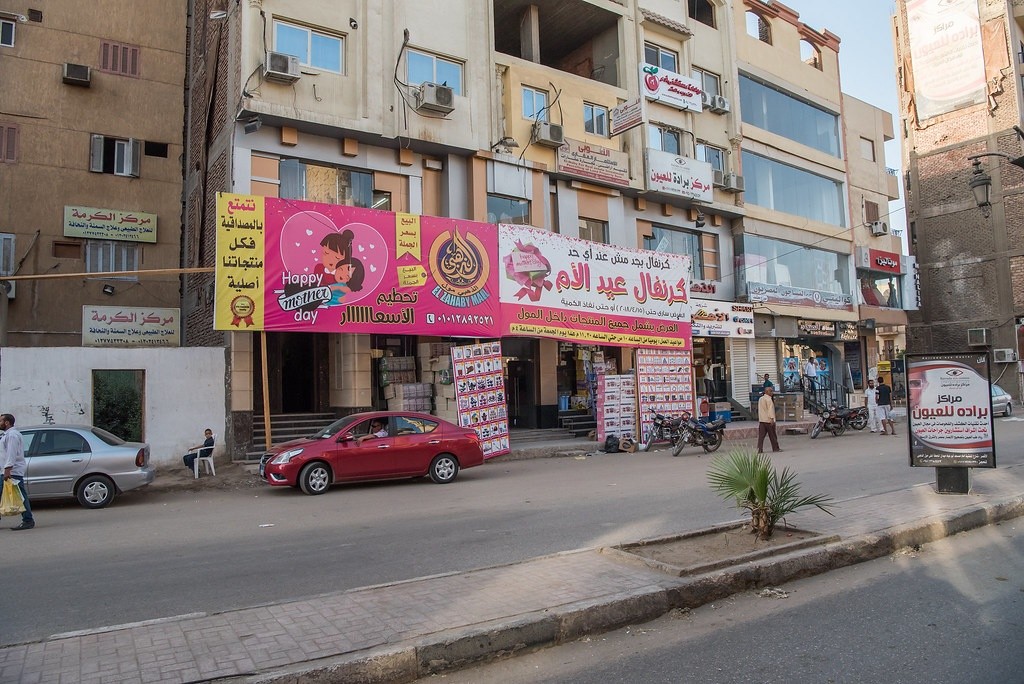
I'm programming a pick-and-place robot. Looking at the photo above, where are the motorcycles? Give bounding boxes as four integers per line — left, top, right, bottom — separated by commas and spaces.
643, 408, 683, 451
829, 398, 870, 430
672, 410, 728, 456
810, 400, 851, 439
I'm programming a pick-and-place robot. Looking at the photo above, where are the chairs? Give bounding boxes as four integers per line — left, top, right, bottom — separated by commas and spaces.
189, 434, 218, 479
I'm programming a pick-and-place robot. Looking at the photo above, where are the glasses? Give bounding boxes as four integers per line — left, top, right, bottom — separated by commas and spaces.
370, 424, 380, 428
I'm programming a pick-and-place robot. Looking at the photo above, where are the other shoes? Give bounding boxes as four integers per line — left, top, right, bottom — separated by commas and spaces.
774, 449, 782, 452
711, 398, 716, 403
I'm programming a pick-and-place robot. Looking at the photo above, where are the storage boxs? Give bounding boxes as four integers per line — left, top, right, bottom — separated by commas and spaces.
699, 401, 732, 425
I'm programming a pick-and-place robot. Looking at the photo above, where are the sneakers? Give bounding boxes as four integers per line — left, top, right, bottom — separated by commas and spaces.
10, 520, 35, 530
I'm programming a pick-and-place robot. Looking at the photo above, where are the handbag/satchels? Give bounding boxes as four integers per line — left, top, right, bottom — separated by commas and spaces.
0, 478, 27, 517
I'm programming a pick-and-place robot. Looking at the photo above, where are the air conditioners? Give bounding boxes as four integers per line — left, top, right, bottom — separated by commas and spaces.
262, 52, 301, 81
62, 62, 92, 85
537, 122, 565, 148
420, 81, 454, 113
701, 90, 711, 106
966, 327, 991, 346
0, 280, 16, 298
872, 221, 889, 235
725, 172, 746, 194
709, 169, 725, 186
712, 95, 730, 115
993, 349, 1014, 364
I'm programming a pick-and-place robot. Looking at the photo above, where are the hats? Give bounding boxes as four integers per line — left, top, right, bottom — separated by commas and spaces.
819, 361, 825, 366
669, 366, 675, 371
472, 413, 479, 422
481, 413, 487, 417
469, 397, 477, 403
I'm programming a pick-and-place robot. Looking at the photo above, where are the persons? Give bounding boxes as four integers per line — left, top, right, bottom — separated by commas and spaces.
909, 370, 929, 408
183, 429, 214, 478
757, 387, 782, 453
356, 419, 388, 447
763, 374, 774, 405
875, 377, 898, 435
805, 357, 817, 396
703, 358, 723, 403
0, 414, 35, 530
864, 379, 886, 433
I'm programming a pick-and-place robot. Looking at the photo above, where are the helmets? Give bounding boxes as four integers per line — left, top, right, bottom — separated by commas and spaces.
681, 412, 690, 421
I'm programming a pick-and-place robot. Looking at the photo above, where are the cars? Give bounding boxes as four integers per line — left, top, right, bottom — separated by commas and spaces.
0, 424, 158, 509
258, 410, 485, 495
990, 384, 1013, 417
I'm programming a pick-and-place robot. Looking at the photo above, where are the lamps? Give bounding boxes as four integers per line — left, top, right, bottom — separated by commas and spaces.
854, 316, 875, 332
492, 135, 518, 152
103, 285, 116, 294
244, 112, 265, 133
965, 150, 1015, 219
691, 202, 705, 228
